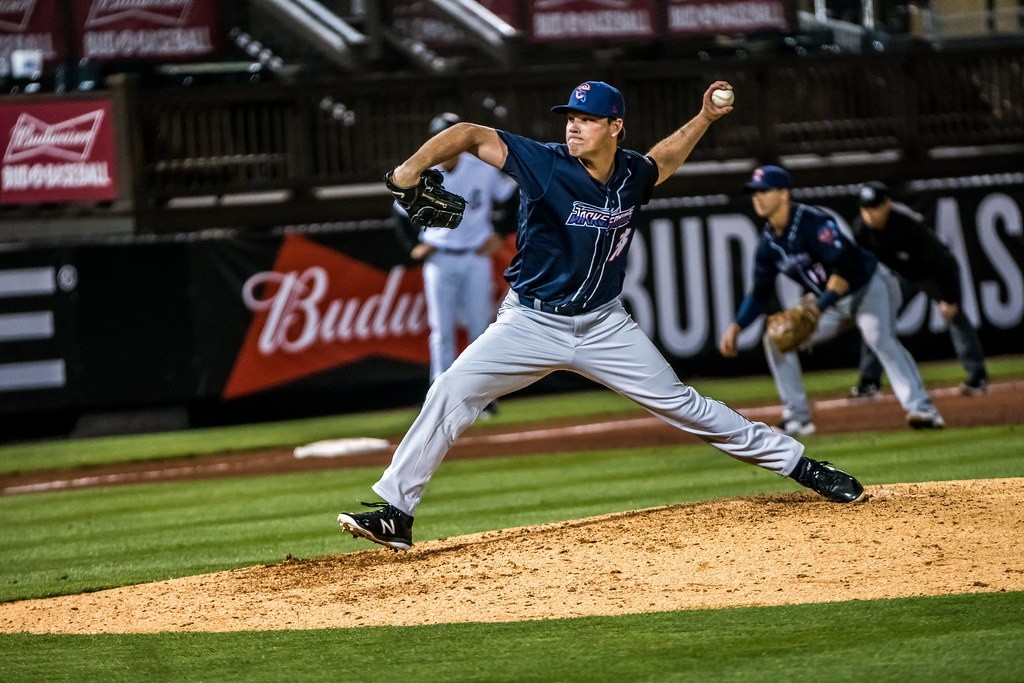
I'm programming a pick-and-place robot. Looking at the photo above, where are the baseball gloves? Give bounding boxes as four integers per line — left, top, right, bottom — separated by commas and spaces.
382, 166, 470, 233
766, 305, 819, 356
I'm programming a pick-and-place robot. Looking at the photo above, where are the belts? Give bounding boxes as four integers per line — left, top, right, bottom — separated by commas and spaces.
435, 247, 468, 256
518, 294, 555, 314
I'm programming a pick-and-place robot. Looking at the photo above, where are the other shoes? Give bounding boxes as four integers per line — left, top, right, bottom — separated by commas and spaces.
960, 370, 989, 396
848, 385, 879, 398
482, 401, 499, 417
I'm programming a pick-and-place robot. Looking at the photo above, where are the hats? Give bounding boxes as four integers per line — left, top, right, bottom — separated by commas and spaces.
859, 180, 888, 208
428, 112, 461, 136
550, 81, 625, 121
743, 166, 790, 190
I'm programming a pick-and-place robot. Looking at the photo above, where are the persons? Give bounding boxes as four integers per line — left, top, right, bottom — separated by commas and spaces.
846, 182, 990, 397
721, 166, 946, 435
338, 81, 865, 552
409, 112, 520, 426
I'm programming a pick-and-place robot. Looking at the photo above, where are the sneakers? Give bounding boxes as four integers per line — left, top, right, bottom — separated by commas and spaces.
338, 499, 415, 552
795, 455, 866, 503
905, 413, 945, 430
770, 418, 817, 435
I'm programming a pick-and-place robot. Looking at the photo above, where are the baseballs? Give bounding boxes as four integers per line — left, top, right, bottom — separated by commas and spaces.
712, 89, 735, 108
